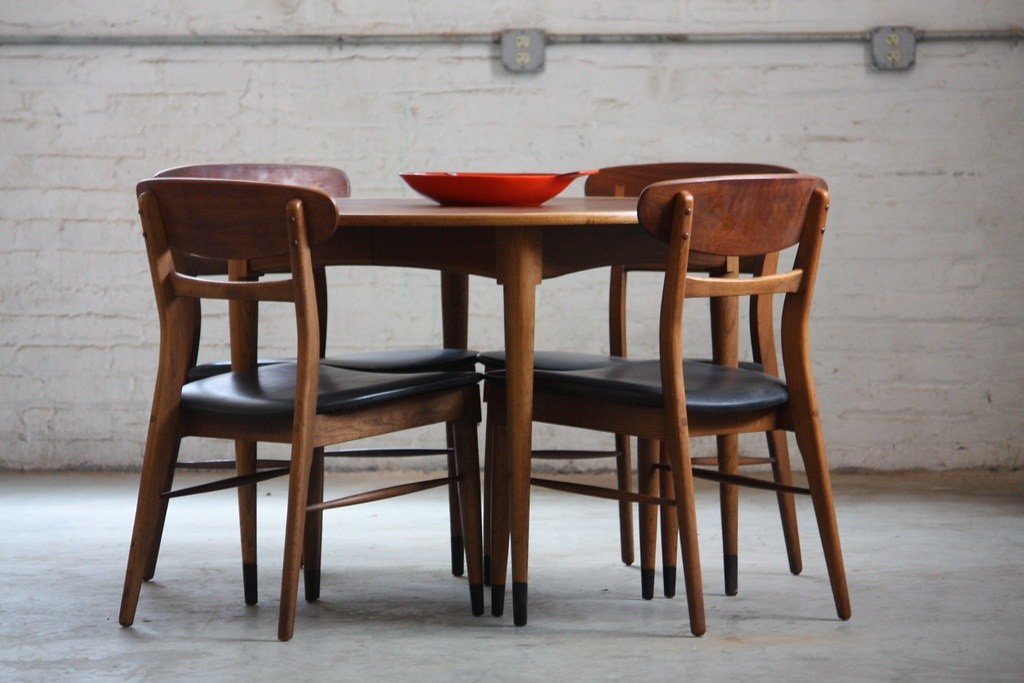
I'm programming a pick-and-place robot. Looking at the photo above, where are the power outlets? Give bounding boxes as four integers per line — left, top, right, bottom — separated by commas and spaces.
500, 27, 546, 74
872, 26, 915, 72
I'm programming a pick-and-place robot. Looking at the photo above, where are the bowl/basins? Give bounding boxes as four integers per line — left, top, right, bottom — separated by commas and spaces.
398, 169, 599, 208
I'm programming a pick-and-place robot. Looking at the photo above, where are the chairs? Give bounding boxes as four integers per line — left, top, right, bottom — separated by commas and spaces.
121, 162, 483, 640
474, 163, 853, 639
154, 161, 482, 608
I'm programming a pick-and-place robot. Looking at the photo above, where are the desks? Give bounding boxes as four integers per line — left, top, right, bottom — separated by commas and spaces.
328, 194, 666, 589
